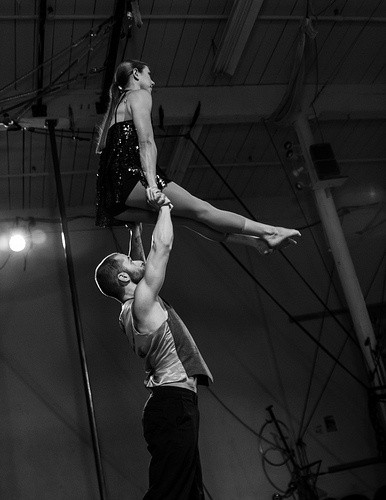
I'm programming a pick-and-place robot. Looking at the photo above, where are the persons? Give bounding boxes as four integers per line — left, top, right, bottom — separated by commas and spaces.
96, 59, 301, 255
95, 187, 213, 500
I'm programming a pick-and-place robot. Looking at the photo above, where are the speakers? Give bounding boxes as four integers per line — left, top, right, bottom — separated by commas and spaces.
309, 143, 341, 179
